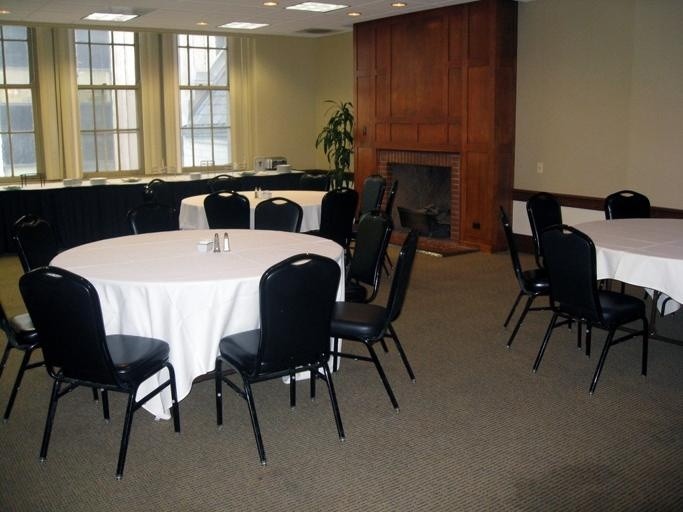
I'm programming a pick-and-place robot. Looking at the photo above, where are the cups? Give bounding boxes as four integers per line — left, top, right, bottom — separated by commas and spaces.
190, 173, 199, 180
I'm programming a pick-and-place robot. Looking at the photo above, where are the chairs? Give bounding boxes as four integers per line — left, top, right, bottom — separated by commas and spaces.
603, 190, 656, 300
18, 265, 181, 481
303, 186, 359, 264
358, 174, 399, 275
532, 224, 648, 395
2, 305, 47, 425
527, 192, 563, 270
345, 208, 395, 353
499, 205, 582, 351
213, 252, 347, 466
12, 214, 59, 272
310, 226, 421, 414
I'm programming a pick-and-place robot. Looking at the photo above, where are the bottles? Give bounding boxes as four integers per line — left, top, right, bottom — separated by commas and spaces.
213, 233, 220, 252
254, 187, 261, 198
223, 232, 229, 252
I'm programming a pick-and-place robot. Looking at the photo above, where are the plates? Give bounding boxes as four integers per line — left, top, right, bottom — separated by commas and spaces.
239, 171, 255, 176
4, 186, 19, 189
121, 178, 139, 182
276, 164, 291, 174
90, 178, 107, 184
63, 179, 82, 186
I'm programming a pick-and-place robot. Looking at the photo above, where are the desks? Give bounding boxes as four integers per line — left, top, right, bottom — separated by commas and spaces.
1, 167, 329, 260
47, 229, 346, 424
